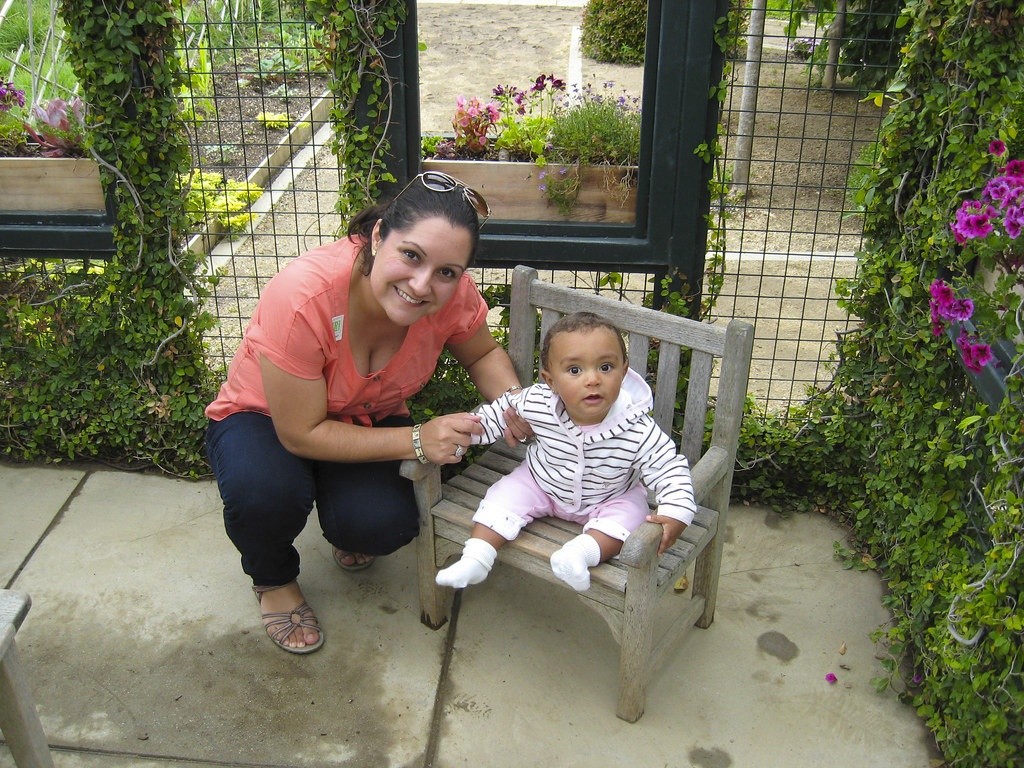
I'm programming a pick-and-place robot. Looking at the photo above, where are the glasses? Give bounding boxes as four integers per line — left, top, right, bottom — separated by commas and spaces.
392, 171, 492, 231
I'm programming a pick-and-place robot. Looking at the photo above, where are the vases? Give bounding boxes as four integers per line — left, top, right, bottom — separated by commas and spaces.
0, 133, 102, 210
421, 135, 637, 221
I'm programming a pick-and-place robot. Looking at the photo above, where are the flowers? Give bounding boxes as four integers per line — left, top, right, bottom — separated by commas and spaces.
433, 70, 642, 216
0, 79, 92, 156
928, 278, 993, 372
949, 140, 1024, 251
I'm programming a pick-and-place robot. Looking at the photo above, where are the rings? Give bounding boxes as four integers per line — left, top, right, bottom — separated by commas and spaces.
517, 435, 528, 444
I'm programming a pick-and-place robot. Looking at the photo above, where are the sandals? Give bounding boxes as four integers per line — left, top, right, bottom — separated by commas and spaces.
332, 544, 376, 572
252, 576, 323, 653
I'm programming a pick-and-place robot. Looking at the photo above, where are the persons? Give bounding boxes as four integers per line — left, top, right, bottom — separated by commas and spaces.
435, 312, 697, 594
204, 171, 536, 654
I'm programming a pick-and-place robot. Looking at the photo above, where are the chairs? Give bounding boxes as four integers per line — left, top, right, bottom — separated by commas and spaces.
399, 266, 754, 723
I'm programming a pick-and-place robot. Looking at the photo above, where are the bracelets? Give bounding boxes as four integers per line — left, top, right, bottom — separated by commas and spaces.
499, 385, 525, 398
412, 423, 430, 466
